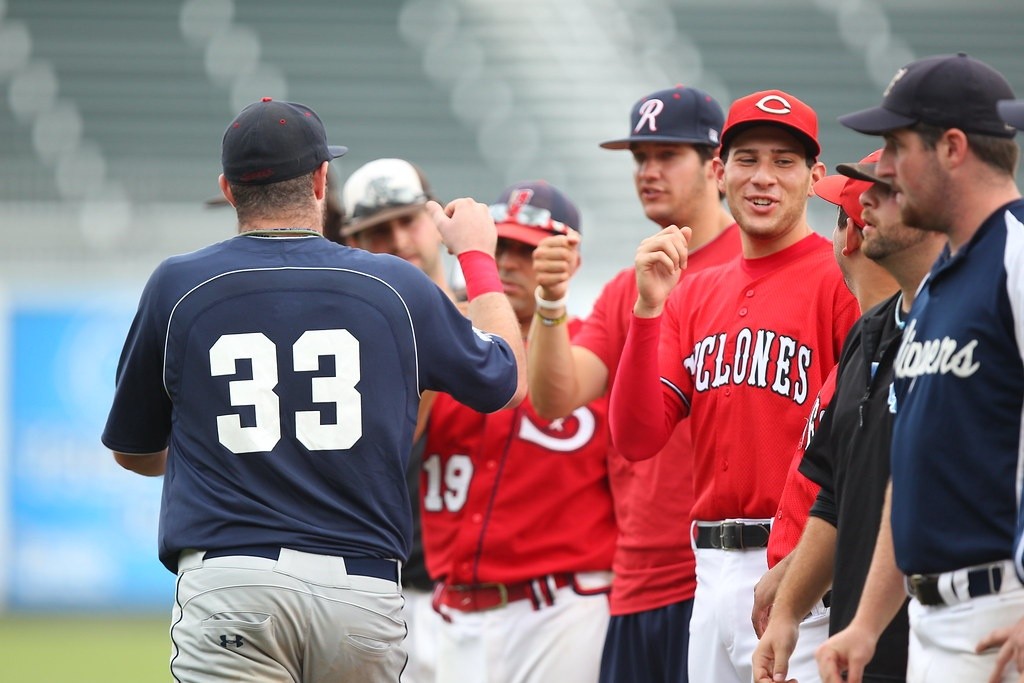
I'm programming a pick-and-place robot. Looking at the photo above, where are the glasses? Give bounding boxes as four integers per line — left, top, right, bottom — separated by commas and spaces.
487, 203, 575, 234
346, 186, 426, 222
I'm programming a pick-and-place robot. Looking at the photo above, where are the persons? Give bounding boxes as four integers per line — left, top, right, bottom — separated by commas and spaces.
314, 160, 616, 683
608, 89, 861, 683
99, 94, 526, 683
527, 87, 743, 683
751, 45, 1024, 682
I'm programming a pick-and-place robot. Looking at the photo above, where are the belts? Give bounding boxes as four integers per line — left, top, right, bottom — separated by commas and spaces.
202, 544, 397, 584
695, 521, 770, 550
802, 588, 833, 620
906, 564, 1022, 605
436, 572, 571, 612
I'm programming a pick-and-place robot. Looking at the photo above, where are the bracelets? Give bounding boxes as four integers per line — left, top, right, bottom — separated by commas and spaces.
536, 309, 567, 326
535, 284, 569, 311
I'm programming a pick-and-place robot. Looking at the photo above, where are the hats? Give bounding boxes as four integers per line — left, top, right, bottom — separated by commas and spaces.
340, 157, 434, 237
494, 180, 583, 247
996, 96, 1024, 132
813, 148, 886, 229
835, 162, 892, 187
718, 90, 820, 162
838, 50, 1018, 139
600, 84, 724, 150
220, 97, 349, 185
